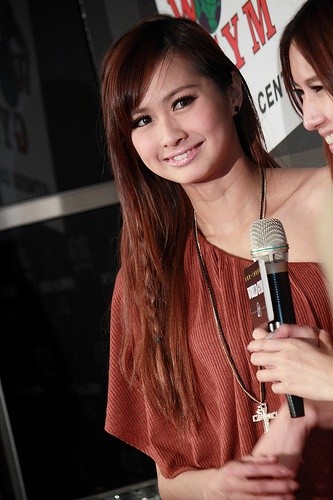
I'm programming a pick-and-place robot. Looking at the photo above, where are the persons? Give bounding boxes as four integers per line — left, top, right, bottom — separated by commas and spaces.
279, 0, 333, 180
100, 13, 333, 500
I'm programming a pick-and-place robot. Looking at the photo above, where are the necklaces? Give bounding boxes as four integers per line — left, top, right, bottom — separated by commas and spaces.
193, 163, 271, 434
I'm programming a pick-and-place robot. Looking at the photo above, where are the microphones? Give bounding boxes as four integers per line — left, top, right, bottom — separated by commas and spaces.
249, 218, 305, 418
